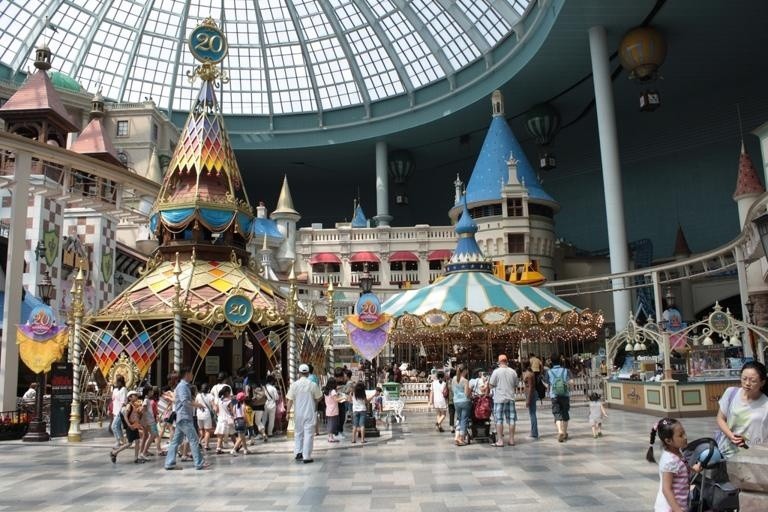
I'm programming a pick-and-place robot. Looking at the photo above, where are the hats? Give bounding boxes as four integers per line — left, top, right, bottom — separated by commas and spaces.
236, 393, 246, 401
299, 364, 309, 373
127, 391, 140, 397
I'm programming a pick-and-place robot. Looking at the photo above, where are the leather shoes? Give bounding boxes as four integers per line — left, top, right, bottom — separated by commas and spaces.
296, 453, 313, 463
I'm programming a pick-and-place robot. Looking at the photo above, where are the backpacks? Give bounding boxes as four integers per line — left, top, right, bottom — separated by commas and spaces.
119, 403, 132, 428
549, 368, 566, 396
252, 387, 266, 406
475, 395, 492, 419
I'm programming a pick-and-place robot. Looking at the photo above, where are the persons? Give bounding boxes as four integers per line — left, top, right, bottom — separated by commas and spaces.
107, 359, 402, 471
645, 416, 702, 511
22, 382, 37, 412
416, 352, 610, 447
714, 360, 768, 458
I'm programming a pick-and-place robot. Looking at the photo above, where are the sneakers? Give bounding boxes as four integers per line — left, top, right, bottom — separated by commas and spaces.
111, 452, 116, 463
216, 449, 253, 455
135, 451, 193, 470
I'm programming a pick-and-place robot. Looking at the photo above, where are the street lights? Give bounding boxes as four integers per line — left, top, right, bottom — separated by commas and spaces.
743, 294, 758, 362
19, 270, 56, 444
353, 261, 380, 438
662, 285, 675, 311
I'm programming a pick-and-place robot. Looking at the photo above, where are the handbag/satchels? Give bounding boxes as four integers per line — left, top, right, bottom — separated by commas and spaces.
443, 387, 448, 398
165, 411, 177, 424
234, 417, 246, 430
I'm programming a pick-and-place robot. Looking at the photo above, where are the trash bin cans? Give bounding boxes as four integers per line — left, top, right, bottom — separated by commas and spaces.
382, 382, 401, 400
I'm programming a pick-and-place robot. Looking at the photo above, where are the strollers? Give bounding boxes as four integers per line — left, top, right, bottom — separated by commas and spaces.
465, 390, 496, 446
680, 437, 742, 512
